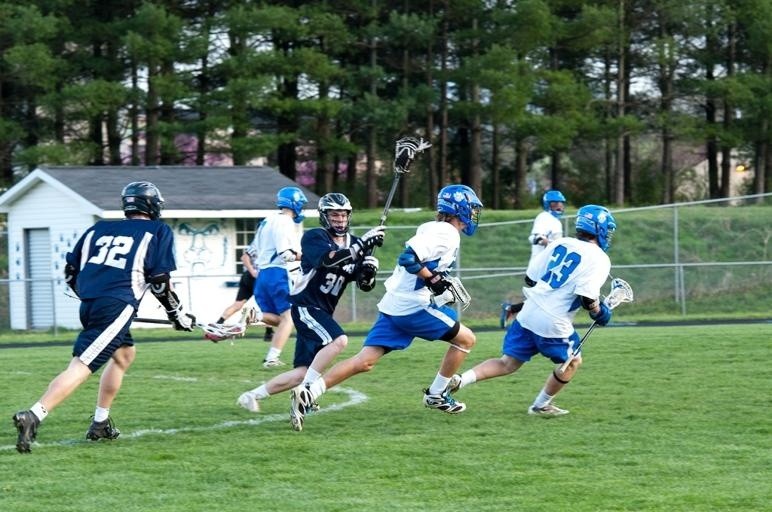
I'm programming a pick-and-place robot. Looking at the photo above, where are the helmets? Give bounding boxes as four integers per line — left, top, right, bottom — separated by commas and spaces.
435, 184, 483, 236
542, 190, 566, 217
575, 204, 618, 253
275, 186, 309, 223
317, 191, 353, 237
120, 181, 164, 220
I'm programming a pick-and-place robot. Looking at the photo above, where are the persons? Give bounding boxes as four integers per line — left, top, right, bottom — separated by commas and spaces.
13, 181, 194, 452
290, 183, 483, 432
500, 190, 567, 329
217, 222, 276, 341
239, 187, 308, 369
445, 204, 617, 413
237, 192, 388, 413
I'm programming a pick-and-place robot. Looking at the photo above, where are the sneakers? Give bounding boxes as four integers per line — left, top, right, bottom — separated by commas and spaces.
11, 409, 41, 455
422, 388, 467, 414
528, 404, 570, 416
85, 415, 121, 440
442, 372, 463, 401
500, 302, 513, 329
239, 307, 256, 338
260, 359, 288, 368
288, 384, 320, 432
236, 392, 260, 414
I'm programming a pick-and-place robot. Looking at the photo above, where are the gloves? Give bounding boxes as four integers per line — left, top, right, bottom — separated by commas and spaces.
355, 255, 379, 293
589, 302, 612, 327
425, 275, 455, 306
167, 303, 197, 333
352, 226, 385, 259
